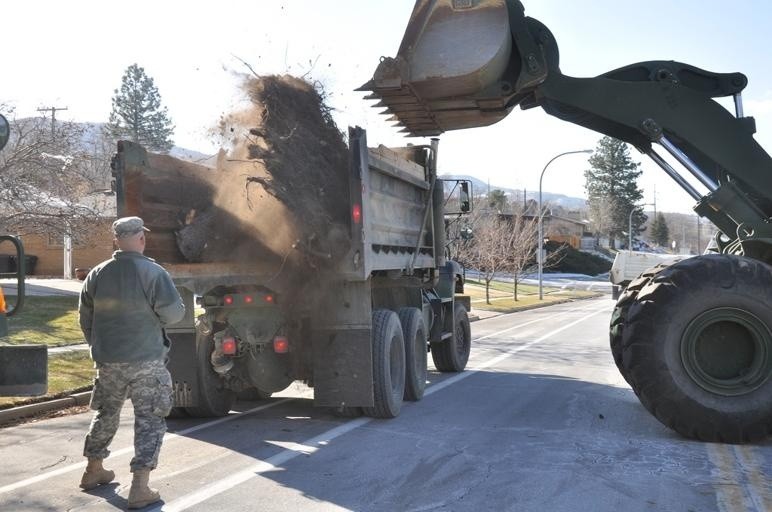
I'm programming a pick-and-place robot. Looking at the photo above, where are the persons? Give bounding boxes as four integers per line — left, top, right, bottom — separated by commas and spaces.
78, 216, 186, 510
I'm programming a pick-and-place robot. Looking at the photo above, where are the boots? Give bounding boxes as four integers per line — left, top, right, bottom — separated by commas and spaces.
127, 468, 160, 509
80, 458, 114, 488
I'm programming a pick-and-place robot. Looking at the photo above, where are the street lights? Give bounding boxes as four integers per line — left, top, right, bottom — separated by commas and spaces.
629, 203, 656, 258
536, 148, 594, 301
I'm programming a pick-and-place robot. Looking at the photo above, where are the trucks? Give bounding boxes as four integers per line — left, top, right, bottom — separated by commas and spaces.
109, 124, 478, 419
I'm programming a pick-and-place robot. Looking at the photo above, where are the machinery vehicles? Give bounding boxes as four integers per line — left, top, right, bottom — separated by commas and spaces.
353, 0, 771, 441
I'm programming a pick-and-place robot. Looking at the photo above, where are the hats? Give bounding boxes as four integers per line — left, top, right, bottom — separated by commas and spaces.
113, 217, 150, 236
